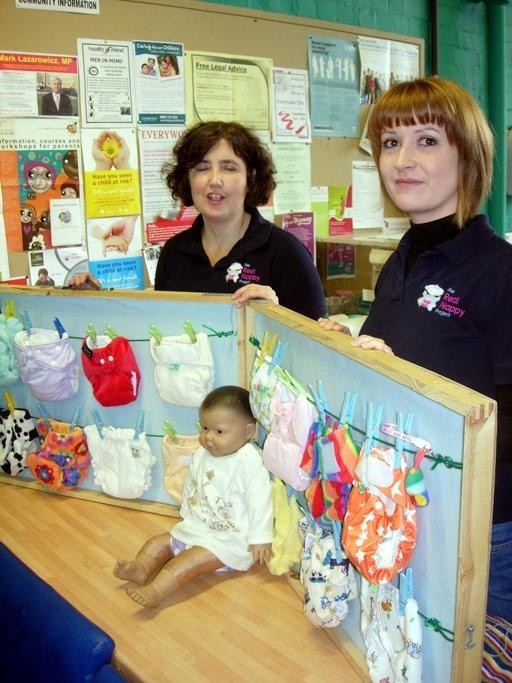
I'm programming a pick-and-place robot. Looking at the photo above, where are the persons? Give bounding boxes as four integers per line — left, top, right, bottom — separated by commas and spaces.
140, 54, 182, 78
318, 77, 511, 621
69, 121, 328, 323
112, 386, 274, 609
97, 215, 139, 256
34, 269, 56, 288
42, 78, 72, 116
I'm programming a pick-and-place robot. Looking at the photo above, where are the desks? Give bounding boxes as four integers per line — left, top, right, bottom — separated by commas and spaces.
317, 228, 405, 250
0, 483, 370, 683
